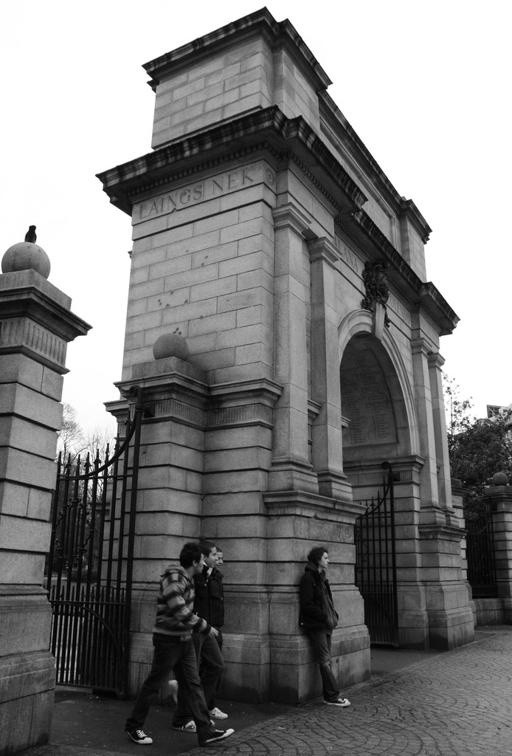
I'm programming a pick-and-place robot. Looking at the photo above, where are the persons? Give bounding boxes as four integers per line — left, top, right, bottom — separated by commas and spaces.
124, 540, 234, 747
298, 547, 352, 708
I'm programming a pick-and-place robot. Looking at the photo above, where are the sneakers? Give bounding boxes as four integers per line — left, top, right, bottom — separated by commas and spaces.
322, 698, 348, 704
128, 728, 153, 744
209, 707, 228, 720
326, 701, 351, 707
171, 720, 197, 733
204, 728, 235, 743
